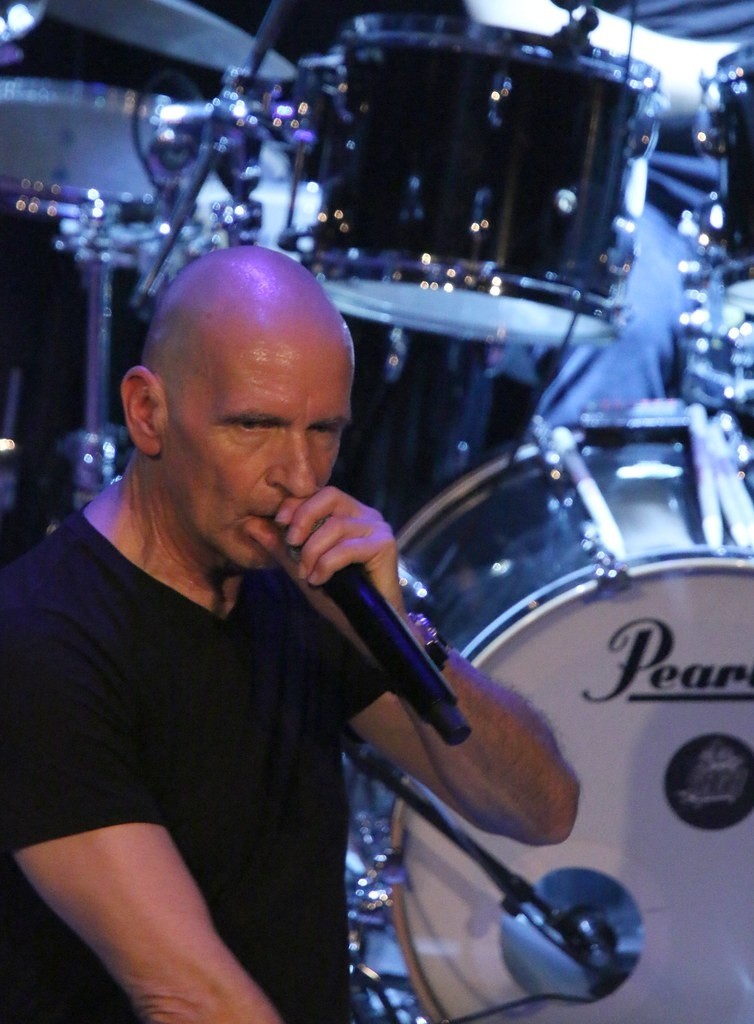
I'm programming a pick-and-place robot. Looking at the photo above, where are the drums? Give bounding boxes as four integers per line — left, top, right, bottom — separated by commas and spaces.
307, 7, 661, 343
707, 44, 754, 309
380, 408, 754, 1024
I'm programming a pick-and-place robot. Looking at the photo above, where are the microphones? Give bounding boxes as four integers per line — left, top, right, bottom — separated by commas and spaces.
276, 514, 471, 746
553, 905, 621, 977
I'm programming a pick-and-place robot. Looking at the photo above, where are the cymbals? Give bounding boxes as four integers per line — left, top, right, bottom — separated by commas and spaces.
38, 0, 299, 83
0, 74, 320, 375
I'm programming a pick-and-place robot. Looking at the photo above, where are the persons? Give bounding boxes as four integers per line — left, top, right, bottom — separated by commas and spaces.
0, 247, 582, 1024
